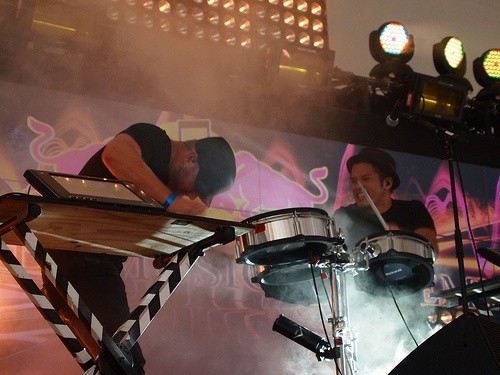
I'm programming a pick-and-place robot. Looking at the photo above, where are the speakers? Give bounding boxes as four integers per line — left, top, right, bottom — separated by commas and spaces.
388, 310, 500, 375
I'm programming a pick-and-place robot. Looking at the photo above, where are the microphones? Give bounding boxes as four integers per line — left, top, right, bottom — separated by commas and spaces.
386, 87, 408, 127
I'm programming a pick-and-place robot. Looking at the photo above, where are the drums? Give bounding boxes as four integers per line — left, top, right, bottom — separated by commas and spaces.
236, 207, 335, 267
251, 262, 327, 288
352, 232, 434, 299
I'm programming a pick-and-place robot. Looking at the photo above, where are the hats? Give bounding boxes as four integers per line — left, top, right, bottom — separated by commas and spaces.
347, 147, 400, 188
194, 137, 236, 208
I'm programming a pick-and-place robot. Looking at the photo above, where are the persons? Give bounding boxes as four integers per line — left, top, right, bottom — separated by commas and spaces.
45, 122, 237, 375
333, 147, 439, 362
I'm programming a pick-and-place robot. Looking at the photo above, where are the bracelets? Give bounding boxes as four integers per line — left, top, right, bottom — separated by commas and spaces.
162, 192, 175, 210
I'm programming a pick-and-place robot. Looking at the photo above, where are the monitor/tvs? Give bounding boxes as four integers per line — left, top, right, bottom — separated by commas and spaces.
24, 169, 165, 210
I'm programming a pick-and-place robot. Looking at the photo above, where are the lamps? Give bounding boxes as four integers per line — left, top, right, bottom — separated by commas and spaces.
369, 21, 415, 78
472, 48, 500, 89
433, 36, 475, 95
